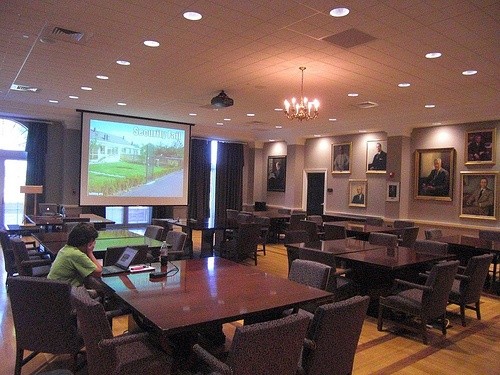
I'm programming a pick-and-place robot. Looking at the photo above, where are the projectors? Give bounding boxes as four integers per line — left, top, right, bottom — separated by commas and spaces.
211, 96, 233, 107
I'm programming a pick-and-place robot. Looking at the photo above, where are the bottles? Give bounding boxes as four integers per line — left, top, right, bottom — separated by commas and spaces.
159, 241, 169, 268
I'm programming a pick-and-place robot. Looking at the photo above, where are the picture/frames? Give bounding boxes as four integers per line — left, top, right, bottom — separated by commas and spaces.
459, 171, 500, 221
366, 140, 387, 174
462, 127, 498, 167
413, 146, 455, 201
266, 155, 287, 192
385, 180, 401, 202
331, 141, 353, 173
348, 178, 368, 208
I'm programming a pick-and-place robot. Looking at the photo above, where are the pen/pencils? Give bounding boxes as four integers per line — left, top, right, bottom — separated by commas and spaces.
131, 267, 144, 270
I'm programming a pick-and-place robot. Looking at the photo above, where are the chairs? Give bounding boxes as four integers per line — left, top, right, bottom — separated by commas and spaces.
192, 208, 500, 375
0, 208, 189, 375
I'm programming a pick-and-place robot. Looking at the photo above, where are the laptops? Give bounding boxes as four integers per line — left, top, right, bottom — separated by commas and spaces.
38, 203, 58, 216
101, 246, 140, 277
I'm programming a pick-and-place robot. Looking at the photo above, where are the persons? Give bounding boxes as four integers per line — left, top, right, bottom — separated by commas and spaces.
368, 143, 386, 170
268, 162, 283, 188
47, 223, 106, 308
351, 186, 364, 204
334, 145, 349, 171
466, 178, 494, 216
467, 135, 490, 161
420, 158, 449, 196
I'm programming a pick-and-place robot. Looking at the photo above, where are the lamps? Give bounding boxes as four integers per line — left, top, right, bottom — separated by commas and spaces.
283, 66, 320, 123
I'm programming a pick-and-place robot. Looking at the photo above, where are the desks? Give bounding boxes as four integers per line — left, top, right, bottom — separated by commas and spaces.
22, 210, 500, 375
